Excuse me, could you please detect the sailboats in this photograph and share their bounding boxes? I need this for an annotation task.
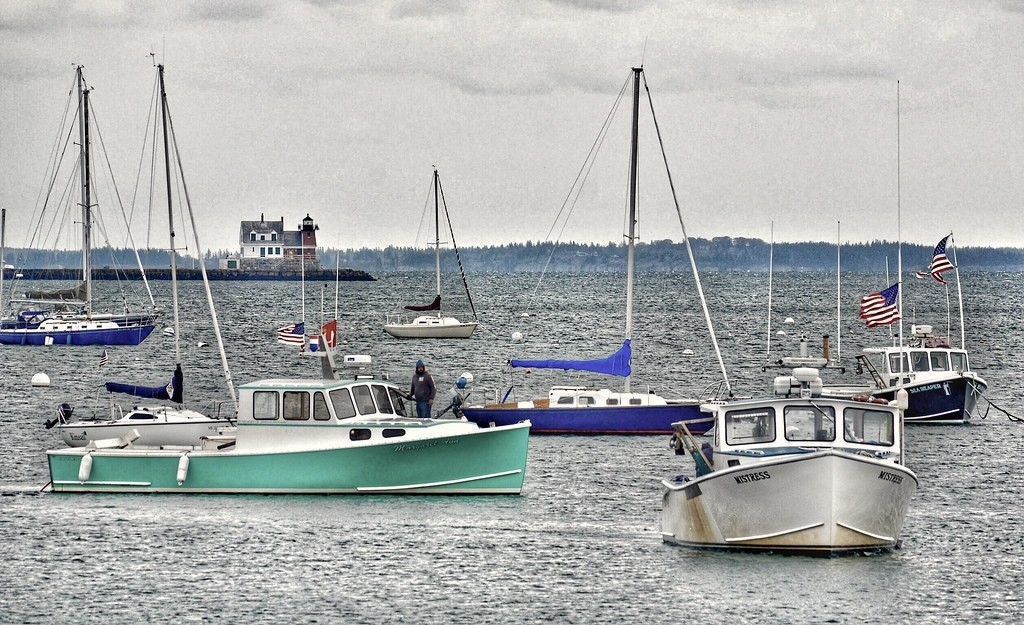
[44,49,535,496]
[383,165,479,339]
[0,62,164,347]
[821,78,988,425]
[298,232,338,358]
[456,34,750,437]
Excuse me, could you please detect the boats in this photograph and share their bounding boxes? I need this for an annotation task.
[661,218,920,562]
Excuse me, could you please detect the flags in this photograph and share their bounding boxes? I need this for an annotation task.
[99,350,108,368]
[322,319,336,348]
[277,322,305,345]
[915,235,956,284]
[860,283,902,328]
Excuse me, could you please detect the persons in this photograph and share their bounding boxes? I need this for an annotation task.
[407,360,436,418]
